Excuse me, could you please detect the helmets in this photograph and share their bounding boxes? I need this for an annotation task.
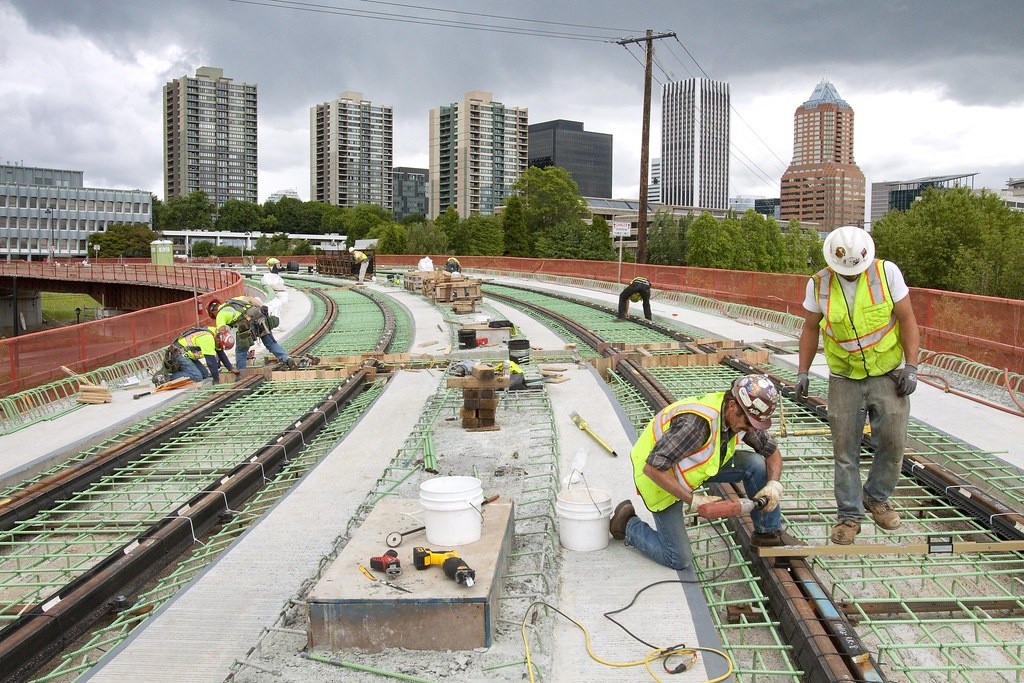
[205,298,221,319]
[733,373,779,430]
[214,330,235,351]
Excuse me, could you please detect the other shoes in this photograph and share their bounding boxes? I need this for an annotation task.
[644,319,653,325]
[612,317,626,322]
[152,368,170,389]
[286,358,300,371]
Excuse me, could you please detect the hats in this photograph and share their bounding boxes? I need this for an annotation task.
[823,226,875,275]
[349,247,355,253]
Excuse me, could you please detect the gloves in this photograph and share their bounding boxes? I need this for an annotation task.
[895,363,919,396]
[795,371,809,404]
[753,480,784,513]
[689,494,723,513]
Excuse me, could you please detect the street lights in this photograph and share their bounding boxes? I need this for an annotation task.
[238,239,243,257]
[74,308,82,324]
[186,242,192,258]
[45,206,55,265]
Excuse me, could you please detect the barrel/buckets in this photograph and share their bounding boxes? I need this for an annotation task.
[555,466,612,552]
[508,340,530,365]
[418,476,485,547]
[458,329,476,350]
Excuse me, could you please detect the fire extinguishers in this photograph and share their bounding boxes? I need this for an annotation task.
[197,296,203,315]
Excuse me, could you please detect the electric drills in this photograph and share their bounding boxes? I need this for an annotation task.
[413,546,477,588]
[698,497,769,521]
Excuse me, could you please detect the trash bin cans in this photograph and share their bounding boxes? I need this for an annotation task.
[150,240,175,266]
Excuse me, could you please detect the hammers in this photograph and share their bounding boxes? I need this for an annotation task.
[133,392,150,400]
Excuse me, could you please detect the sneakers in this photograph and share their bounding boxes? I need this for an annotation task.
[862,498,900,530]
[608,499,636,540]
[830,519,861,545]
[749,525,810,560]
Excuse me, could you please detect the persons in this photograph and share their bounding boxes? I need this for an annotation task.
[610,374,809,571]
[349,247,369,285]
[153,327,241,386]
[266,257,280,274]
[206,296,298,371]
[445,253,462,272]
[611,277,653,322]
[794,226,921,545]
